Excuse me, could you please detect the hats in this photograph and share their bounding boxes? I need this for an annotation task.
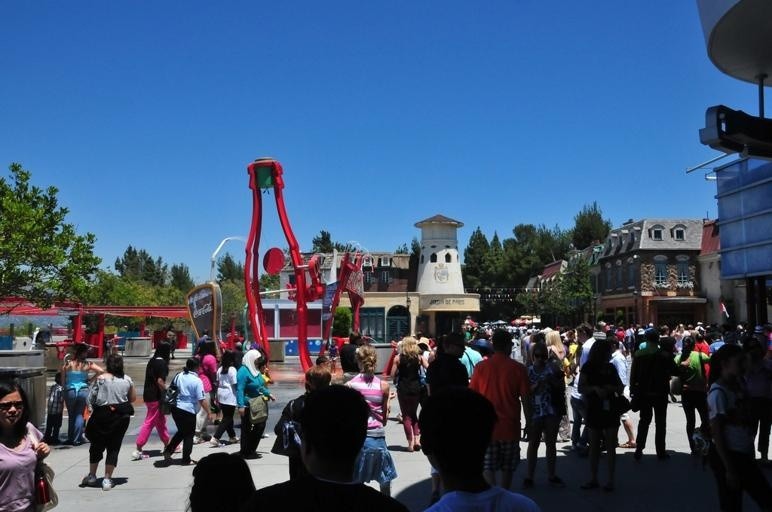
[418,337,432,351]
[697,322,703,324]
[34,439,58,512]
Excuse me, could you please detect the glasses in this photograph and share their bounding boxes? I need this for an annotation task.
[0,402,24,410]
[535,353,548,359]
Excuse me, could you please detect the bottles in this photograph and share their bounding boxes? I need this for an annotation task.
[36,473,49,504]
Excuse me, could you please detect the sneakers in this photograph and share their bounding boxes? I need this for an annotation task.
[521,479,534,489]
[261,433,269,437]
[658,455,671,459]
[228,436,241,444]
[210,437,225,447]
[549,475,562,483]
[131,452,148,461]
[102,479,114,490]
[163,445,181,453]
[82,474,97,485]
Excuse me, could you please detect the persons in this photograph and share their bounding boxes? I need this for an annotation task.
[0,374,50,512]
[183,451,259,511]
[257,313,771,511]
[78,355,137,491]
[30,322,257,464]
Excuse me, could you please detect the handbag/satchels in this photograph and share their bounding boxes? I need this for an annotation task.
[270,399,296,457]
[163,372,181,409]
[670,377,681,395]
[692,389,728,457]
[586,396,630,418]
[248,395,268,423]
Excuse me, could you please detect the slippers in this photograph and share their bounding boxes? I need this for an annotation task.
[620,442,637,448]
